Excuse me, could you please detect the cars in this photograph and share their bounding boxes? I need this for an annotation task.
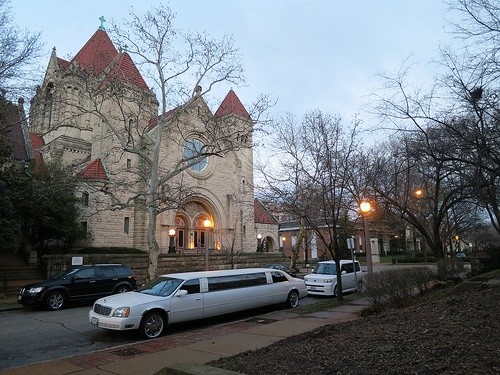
[303,260,363,298]
[265,264,296,282]
[88,268,308,339]
[454,252,467,262]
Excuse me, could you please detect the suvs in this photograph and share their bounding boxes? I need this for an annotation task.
[17,264,138,311]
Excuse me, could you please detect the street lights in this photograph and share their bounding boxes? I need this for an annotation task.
[204,220,211,271]
[282,236,286,254]
[359,201,374,296]
